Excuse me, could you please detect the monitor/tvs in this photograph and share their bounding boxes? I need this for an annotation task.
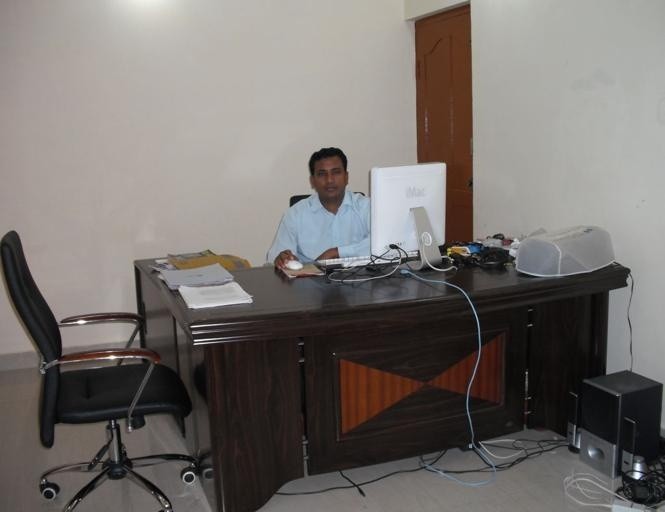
[369,161,448,271]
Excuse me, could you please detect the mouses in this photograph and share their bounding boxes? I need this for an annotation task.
[284,259,304,271]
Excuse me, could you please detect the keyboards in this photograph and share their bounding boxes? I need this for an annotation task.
[317,256,399,267]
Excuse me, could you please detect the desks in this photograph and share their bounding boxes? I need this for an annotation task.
[133,257,629,512]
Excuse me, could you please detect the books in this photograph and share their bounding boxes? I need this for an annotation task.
[150,244,254,309]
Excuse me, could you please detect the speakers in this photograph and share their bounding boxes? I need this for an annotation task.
[566,391,581,453]
[621,416,649,481]
[579,369,664,478]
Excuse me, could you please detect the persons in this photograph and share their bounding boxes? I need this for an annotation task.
[265,147,371,268]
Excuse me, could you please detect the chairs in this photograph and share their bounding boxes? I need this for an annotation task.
[0,230,198,512]
[290,193,365,209]
